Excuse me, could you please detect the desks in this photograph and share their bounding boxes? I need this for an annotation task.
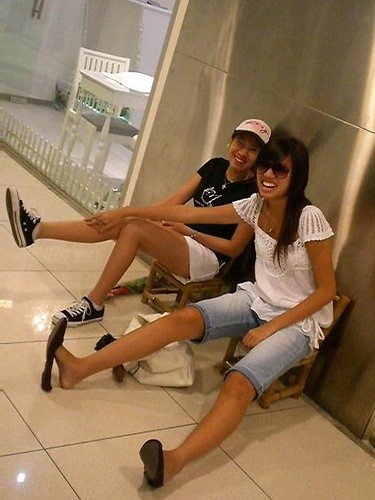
[64,69,150,174]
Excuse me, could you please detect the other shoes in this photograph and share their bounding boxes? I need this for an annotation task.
[40,317,68,392]
[139,439,164,488]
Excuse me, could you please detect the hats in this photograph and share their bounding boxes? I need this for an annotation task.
[231,118,272,145]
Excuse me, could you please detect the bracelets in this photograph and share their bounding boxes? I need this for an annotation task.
[190,231,198,237]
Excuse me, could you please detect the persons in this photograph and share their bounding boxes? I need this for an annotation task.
[41,136,336,488]
[6,118,272,327]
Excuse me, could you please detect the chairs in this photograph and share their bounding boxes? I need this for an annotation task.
[58,46,138,169]
[140,257,237,315]
[221,294,351,409]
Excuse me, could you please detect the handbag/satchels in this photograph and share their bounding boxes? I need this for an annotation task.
[120,311,196,388]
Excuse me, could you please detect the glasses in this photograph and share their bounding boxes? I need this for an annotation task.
[253,161,289,179]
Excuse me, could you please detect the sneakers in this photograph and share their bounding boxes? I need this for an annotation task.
[6,187,42,249]
[52,296,105,328]
